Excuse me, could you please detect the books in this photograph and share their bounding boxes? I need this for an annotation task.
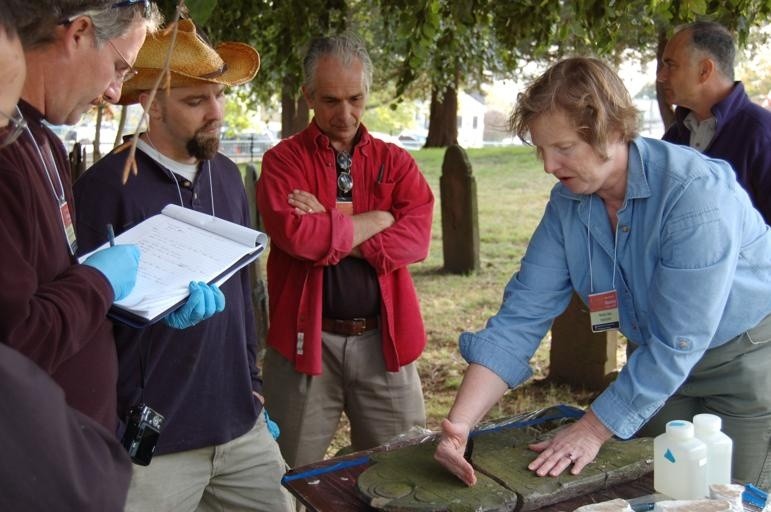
[78,201,271,330]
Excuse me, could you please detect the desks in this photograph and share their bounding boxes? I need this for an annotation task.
[280,406,654,512]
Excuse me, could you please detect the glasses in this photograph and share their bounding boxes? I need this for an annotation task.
[55,12,143,87]
[335,149,355,194]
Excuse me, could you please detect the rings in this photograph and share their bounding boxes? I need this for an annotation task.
[564,452,576,463]
[308,208,314,213]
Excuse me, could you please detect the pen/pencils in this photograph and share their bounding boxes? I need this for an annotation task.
[107,223,115,247]
[377,164,384,184]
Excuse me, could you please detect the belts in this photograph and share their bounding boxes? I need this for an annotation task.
[323,316,378,336]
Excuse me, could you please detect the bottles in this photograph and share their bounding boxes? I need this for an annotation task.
[653,419,709,501]
[692,413,733,485]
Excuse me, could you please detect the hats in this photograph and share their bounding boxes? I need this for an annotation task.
[102,15,264,108]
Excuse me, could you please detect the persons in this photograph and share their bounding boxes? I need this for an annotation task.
[0,0,227,447]
[73,18,299,511]
[659,21,771,228]
[254,32,434,467]
[0,11,29,130]
[435,58,770,495]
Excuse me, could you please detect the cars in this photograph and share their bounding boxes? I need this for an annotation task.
[397,129,428,146]
[220,126,274,158]
[64,128,125,163]
